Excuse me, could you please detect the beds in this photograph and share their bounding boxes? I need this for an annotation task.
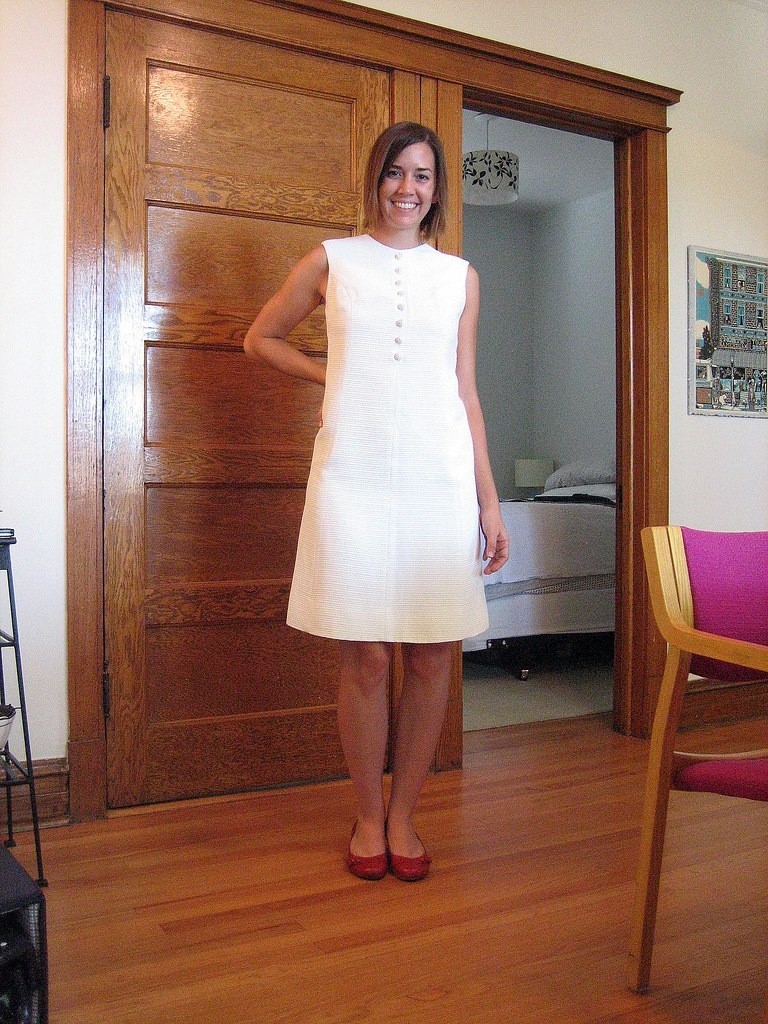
[462,457,616,682]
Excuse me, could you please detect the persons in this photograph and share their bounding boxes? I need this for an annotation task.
[243,121,511,883]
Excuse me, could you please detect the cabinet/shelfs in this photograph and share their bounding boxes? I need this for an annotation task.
[0,528,49,888]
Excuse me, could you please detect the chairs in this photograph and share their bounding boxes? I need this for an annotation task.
[623,524,768,993]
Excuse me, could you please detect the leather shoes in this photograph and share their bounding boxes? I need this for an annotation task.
[383,815,440,882]
[347,822,389,879]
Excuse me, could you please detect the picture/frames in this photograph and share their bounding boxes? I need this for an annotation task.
[686,244,768,419]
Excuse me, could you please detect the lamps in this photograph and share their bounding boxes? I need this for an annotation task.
[462,113,519,206]
[515,459,553,497]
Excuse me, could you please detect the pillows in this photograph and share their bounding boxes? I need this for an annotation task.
[544,458,616,492]
